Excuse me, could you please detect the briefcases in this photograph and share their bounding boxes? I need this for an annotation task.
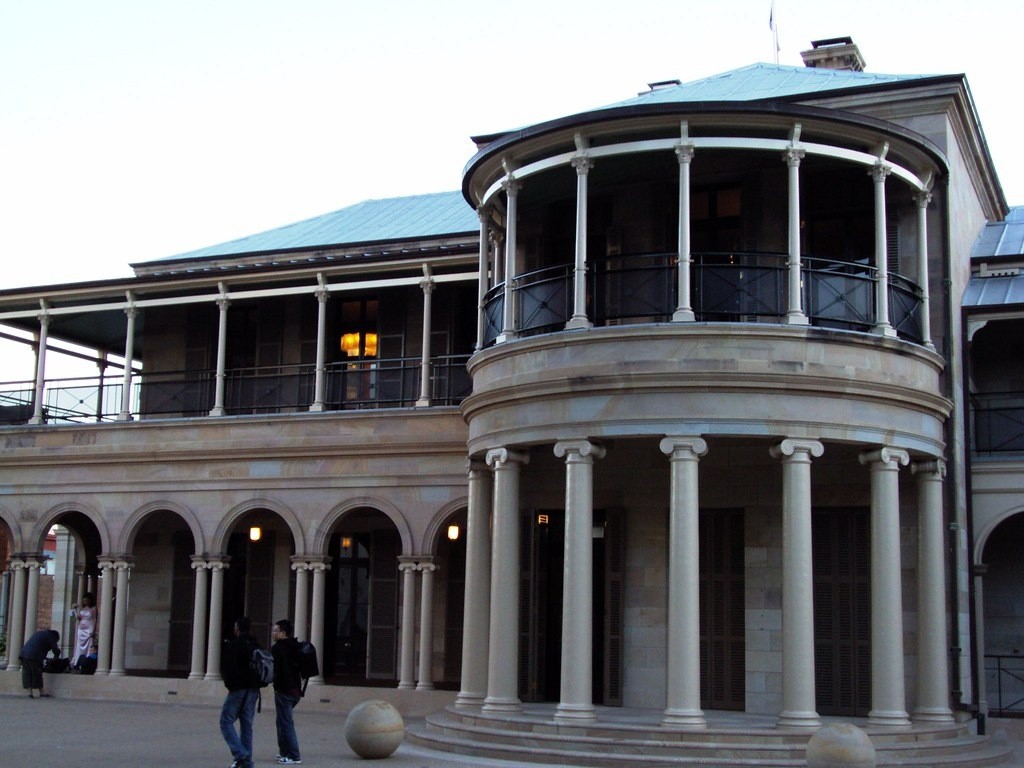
[45,658,71,673]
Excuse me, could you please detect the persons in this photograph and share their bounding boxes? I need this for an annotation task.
[269,618,320,765]
[18,629,61,699]
[219,617,274,768]
[71,585,117,675]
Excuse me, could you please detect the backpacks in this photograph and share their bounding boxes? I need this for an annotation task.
[295,639,319,697]
[240,638,275,713]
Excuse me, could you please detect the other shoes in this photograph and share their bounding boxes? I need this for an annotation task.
[28,695,33,698]
[274,755,282,760]
[279,757,301,764]
[230,753,251,768]
[40,693,53,697]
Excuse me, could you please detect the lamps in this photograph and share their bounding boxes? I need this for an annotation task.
[341,332,379,365]
